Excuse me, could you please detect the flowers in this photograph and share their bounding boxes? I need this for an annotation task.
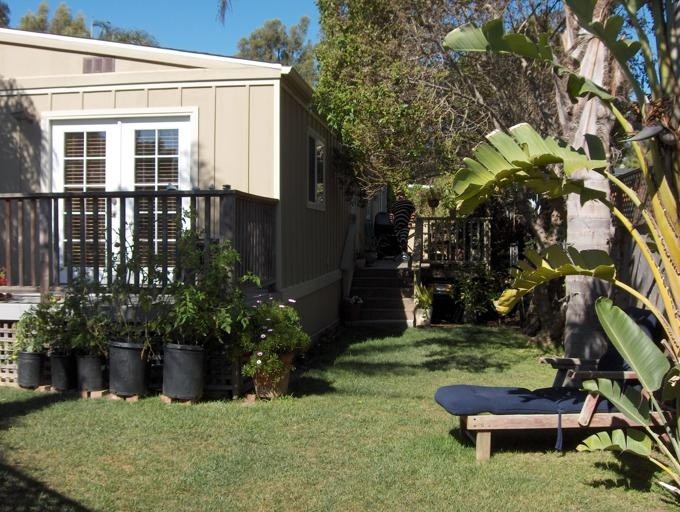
[239,292,311,383]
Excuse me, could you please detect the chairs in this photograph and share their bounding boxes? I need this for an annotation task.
[434,303,673,460]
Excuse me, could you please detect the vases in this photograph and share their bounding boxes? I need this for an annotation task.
[250,349,294,399]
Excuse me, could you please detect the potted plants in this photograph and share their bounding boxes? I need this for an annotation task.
[2,306,50,389]
[426,187,443,207]
[62,245,120,391]
[414,282,432,327]
[102,218,173,396]
[153,206,262,401]
[39,284,84,391]
